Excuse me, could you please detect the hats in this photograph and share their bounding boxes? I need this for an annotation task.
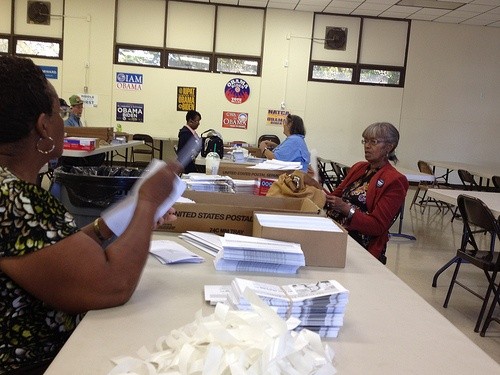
[69,95,83,105]
[59,98,70,107]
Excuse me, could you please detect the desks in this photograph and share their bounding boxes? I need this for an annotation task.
[58,139,148,165]
[426,189,500,223]
[316,152,437,240]
[36,219,499,375]
[194,147,255,165]
[427,158,500,193]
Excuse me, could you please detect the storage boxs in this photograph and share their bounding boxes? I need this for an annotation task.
[158,140,352,268]
[63,126,129,152]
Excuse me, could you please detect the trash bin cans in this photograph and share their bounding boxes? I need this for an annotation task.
[54,165,144,247]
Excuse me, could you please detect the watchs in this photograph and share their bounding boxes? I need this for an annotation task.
[348,205,356,216]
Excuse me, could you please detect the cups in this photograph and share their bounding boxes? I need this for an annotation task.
[205,152,220,175]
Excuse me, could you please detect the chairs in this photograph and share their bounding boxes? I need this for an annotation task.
[130,133,153,164]
[408,160,500,341]
[257,135,282,148]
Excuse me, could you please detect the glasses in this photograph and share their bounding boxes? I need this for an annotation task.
[362,139,389,145]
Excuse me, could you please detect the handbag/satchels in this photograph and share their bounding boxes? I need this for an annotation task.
[266,172,327,209]
[200,136,223,159]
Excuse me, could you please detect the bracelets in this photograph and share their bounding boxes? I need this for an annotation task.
[94,218,111,243]
[262,147,268,155]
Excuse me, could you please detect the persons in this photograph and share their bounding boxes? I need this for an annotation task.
[322,122,409,260]
[176,110,206,174]
[0,54,184,375]
[259,114,310,174]
[47,95,106,172]
